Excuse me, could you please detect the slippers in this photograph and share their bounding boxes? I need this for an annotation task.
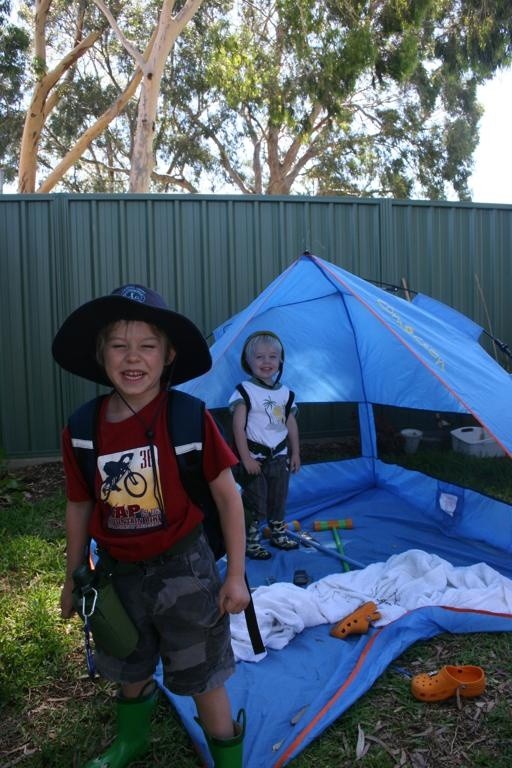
[329,601,383,640]
[410,662,487,703]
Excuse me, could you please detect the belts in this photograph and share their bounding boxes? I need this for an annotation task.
[156,518,205,557]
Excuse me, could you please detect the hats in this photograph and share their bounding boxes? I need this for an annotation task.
[47,279,216,394]
[239,330,286,378]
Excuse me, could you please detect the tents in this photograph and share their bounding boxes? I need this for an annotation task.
[81,247,510,768]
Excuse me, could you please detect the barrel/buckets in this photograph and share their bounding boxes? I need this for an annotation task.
[402,428,424,455]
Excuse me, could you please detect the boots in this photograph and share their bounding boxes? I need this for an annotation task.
[80,689,161,768]
[243,517,272,561]
[267,518,301,551]
[194,716,245,768]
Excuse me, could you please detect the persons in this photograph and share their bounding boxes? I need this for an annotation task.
[226,329,301,561]
[49,280,254,768]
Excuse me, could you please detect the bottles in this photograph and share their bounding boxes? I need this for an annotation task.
[70,565,140,661]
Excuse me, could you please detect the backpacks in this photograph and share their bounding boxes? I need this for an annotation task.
[65,388,252,565]
